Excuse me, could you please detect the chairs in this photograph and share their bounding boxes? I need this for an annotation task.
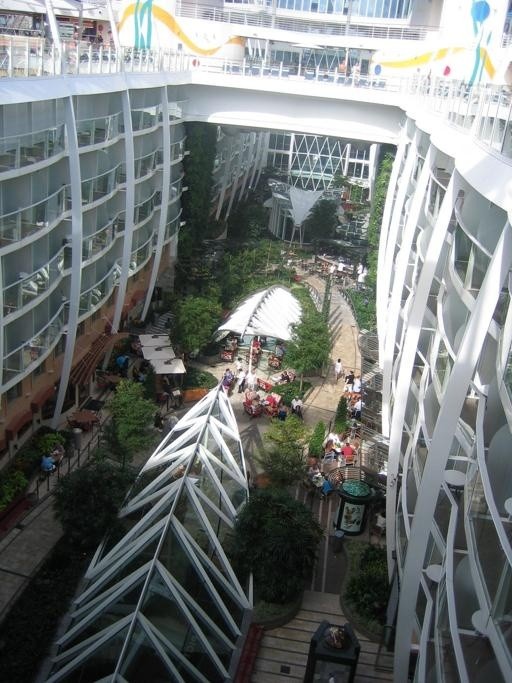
[305,370,360,485]
[221,335,303,421]
[36,353,134,481]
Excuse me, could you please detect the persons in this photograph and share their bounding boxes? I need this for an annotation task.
[339,61,361,84]
[154,411,178,432]
[356,262,364,275]
[220,335,304,424]
[40,441,65,472]
[166,318,172,329]
[321,261,346,274]
[349,423,360,439]
[309,464,332,497]
[117,354,147,382]
[335,359,365,419]
[325,439,355,459]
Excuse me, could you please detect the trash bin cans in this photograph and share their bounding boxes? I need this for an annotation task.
[333,531,344,553]
[73,428,82,450]
